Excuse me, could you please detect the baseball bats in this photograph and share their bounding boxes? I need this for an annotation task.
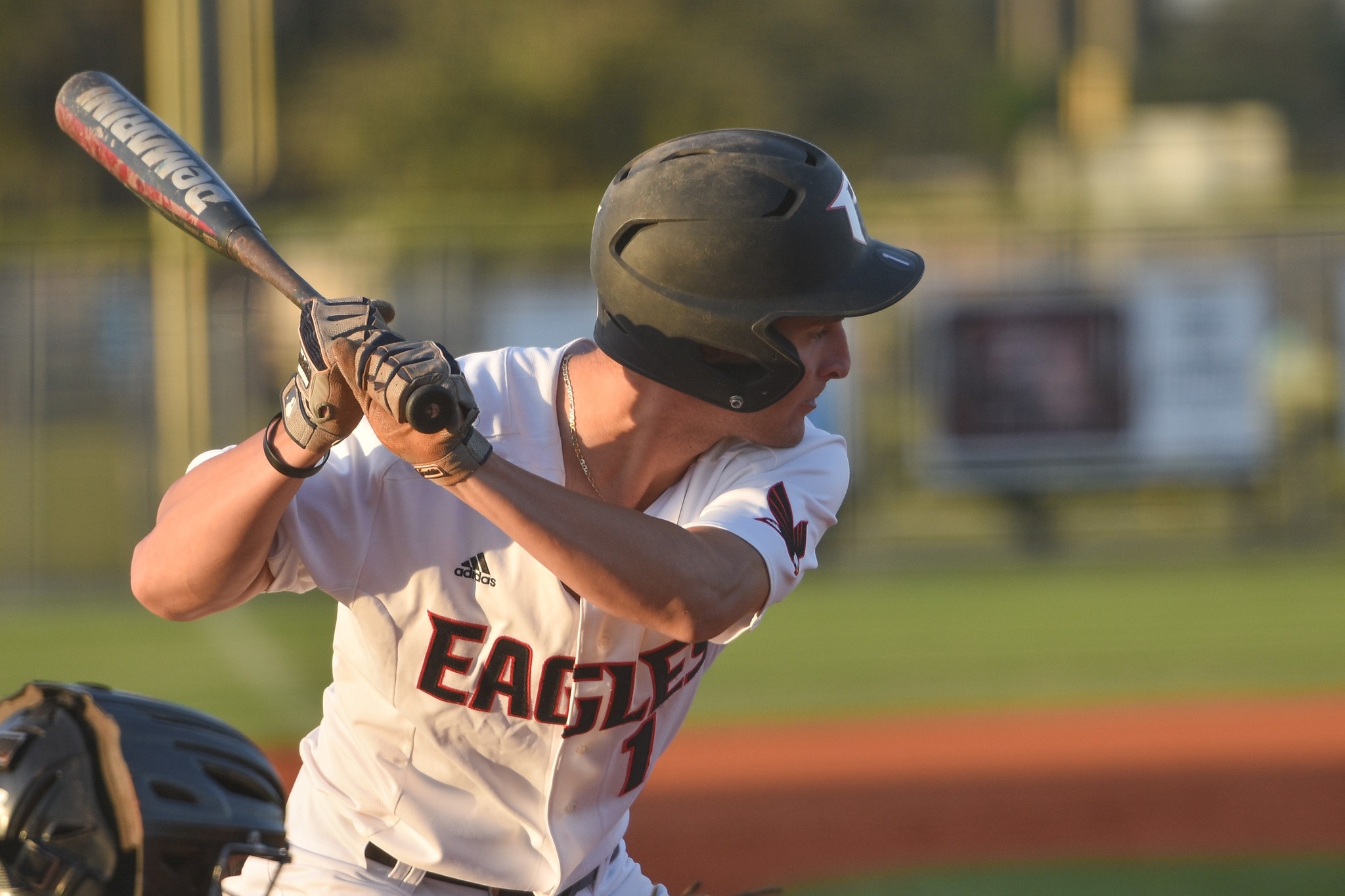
[55,70,456,434]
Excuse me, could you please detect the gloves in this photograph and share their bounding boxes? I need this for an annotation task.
[282,296,405,455]
[331,327,502,491]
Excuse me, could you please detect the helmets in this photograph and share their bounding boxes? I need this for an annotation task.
[0,672,289,896]
[587,130,924,414]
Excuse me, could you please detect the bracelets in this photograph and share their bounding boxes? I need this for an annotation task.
[261,410,331,478]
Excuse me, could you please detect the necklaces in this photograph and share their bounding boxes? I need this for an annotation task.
[562,352,610,501]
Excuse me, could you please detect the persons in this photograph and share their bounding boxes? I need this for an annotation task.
[130,129,927,896]
